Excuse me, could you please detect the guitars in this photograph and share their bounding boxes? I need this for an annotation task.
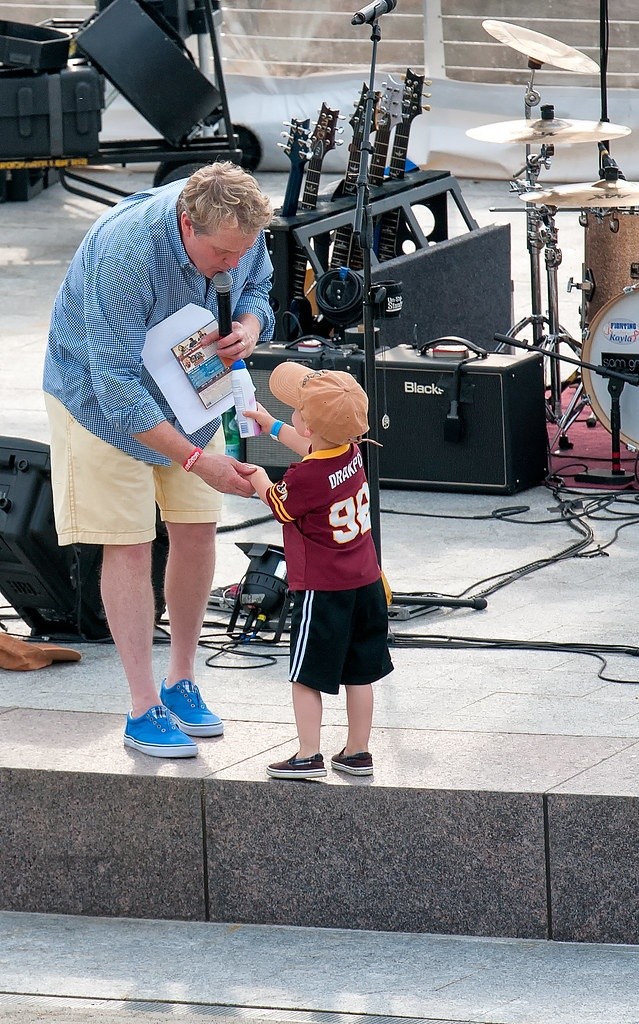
[275,67,433,320]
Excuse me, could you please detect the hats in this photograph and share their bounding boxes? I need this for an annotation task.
[269,362,370,445]
[0,632,81,671]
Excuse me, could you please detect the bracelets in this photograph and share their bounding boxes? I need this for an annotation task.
[270,420,284,443]
[183,447,204,473]
[178,330,209,369]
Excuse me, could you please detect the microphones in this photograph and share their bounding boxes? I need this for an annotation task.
[213,272,233,339]
[351,0,397,25]
[444,369,462,442]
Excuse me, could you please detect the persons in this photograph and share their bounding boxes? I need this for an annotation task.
[237,361,395,779]
[43,161,278,758]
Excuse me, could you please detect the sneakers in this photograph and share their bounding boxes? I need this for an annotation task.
[159,677,224,737]
[331,747,373,776]
[124,706,198,757]
[266,751,327,779]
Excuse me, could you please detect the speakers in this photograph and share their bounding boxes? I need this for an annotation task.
[0,434,169,641]
[240,340,549,494]
[74,0,223,148]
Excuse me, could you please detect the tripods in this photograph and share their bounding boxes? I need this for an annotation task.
[494,53,586,423]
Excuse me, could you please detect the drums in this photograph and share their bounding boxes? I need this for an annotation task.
[581,205,639,331]
[580,282,639,453]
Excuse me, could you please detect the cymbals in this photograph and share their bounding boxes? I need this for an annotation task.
[481,18,604,75]
[517,181,639,208]
[462,117,634,146]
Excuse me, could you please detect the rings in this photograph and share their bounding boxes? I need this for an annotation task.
[239,341,246,348]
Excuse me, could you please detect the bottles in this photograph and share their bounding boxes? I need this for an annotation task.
[231,359,260,438]
